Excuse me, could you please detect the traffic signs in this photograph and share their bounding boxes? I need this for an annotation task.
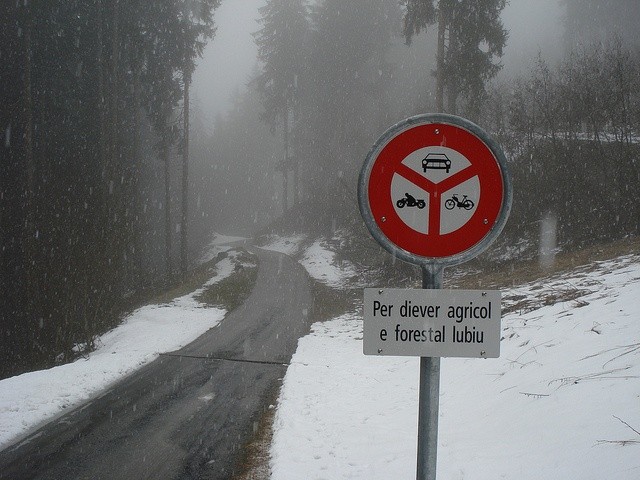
[363,288,501,358]
